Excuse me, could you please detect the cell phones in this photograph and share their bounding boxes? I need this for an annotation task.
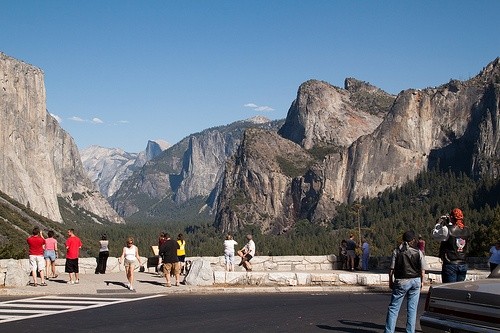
[440,215,447,220]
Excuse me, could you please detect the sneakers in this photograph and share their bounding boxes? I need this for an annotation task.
[41,282,47,286]
[67,280,74,285]
[74,279,80,284]
[34,283,37,287]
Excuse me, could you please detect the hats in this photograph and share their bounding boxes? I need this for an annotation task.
[418,234,422,238]
[494,239,500,244]
[402,231,415,242]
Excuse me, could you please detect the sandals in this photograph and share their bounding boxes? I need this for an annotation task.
[52,274,58,278]
[46,277,49,280]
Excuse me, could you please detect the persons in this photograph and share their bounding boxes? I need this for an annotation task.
[223,235,238,272]
[346,234,357,271]
[44,231,58,280]
[159,232,166,250]
[339,240,348,270]
[432,208,470,283]
[384,231,425,333]
[95,234,110,274]
[362,239,370,271]
[237,234,255,271]
[119,238,143,291]
[26,228,48,287]
[417,234,425,255]
[488,240,500,274]
[177,233,187,275]
[65,229,83,284]
[159,233,180,287]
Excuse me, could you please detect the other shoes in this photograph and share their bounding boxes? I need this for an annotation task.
[239,258,248,266]
[341,265,370,275]
[175,282,179,286]
[166,283,171,287]
[128,283,133,290]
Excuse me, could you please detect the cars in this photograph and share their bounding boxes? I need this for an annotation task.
[418,264,500,333]
